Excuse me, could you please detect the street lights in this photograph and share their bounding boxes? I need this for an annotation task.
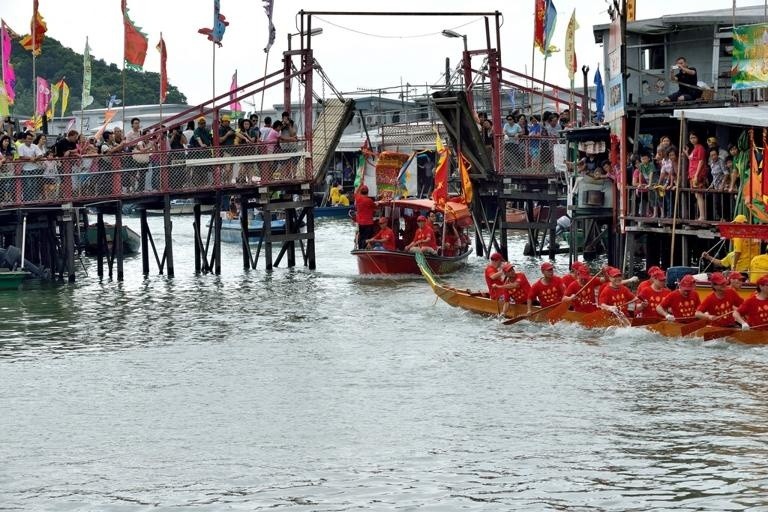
[283,26,326,141]
[438,28,472,107]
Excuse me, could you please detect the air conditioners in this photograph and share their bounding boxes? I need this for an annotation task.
[365,115,377,126]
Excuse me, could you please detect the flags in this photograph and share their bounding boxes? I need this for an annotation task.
[156,41,168,104]
[564,9,576,77]
[198,1,230,46]
[262,0,277,52]
[20,7,49,57]
[229,74,241,112]
[120,1,150,73]
[430,132,472,204]
[83,45,94,107]
[535,1,559,53]
[34,76,69,113]
[2,23,17,105]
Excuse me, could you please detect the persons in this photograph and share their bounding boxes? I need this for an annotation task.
[1,111,302,195]
[330,180,470,255]
[478,56,768,333]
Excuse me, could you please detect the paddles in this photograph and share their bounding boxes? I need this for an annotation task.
[631,317,697,327]
[583,296,638,330]
[503,302,563,327]
[681,311,733,337]
[704,323,768,342]
[548,271,602,326]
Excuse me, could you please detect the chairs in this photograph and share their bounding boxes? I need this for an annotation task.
[659,89,712,106]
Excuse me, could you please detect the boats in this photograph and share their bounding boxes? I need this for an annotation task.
[0,216,38,292]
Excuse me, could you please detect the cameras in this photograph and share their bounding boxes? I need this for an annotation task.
[175,127,182,134]
[288,117,294,125]
[672,66,679,69]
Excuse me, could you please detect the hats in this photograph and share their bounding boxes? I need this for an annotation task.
[490,253,768,290]
[706,136,717,145]
[196,113,234,124]
[360,185,426,225]
